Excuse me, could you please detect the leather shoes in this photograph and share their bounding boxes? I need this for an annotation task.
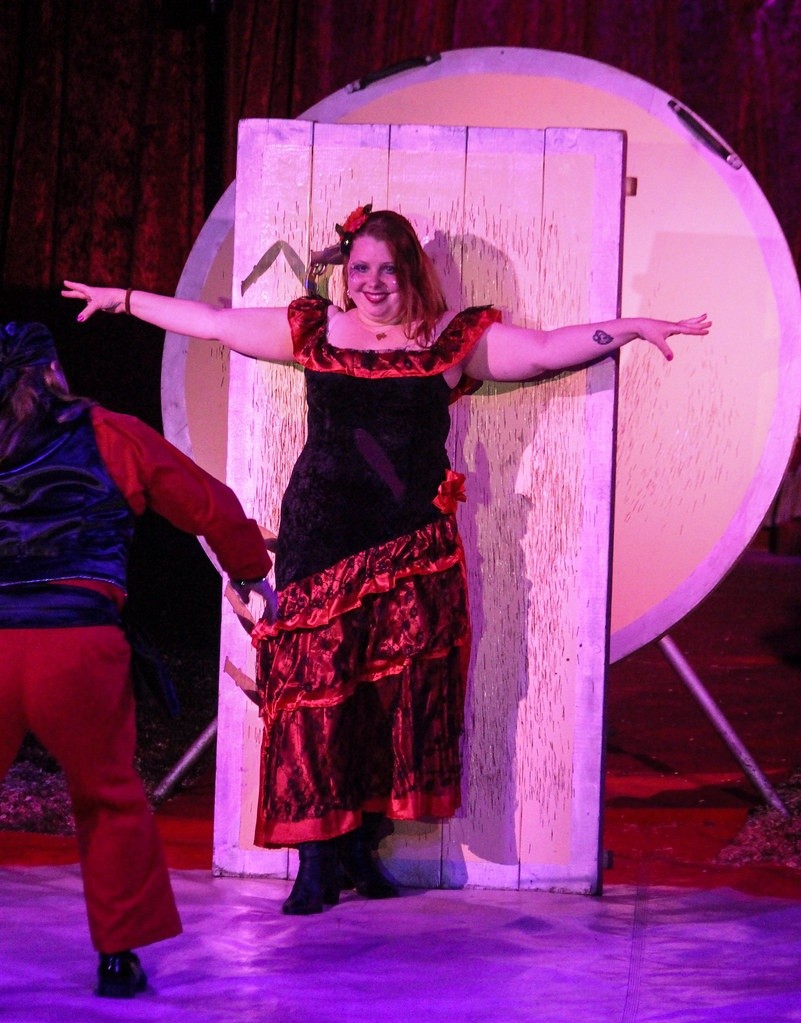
[96,952,147,998]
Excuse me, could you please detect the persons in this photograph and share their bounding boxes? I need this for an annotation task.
[60,203,713,916]
[0,323,272,995]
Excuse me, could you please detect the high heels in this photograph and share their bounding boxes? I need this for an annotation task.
[344,827,397,899]
[282,838,343,914]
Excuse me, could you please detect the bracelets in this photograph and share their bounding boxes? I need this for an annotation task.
[239,577,266,586]
[125,288,132,315]
